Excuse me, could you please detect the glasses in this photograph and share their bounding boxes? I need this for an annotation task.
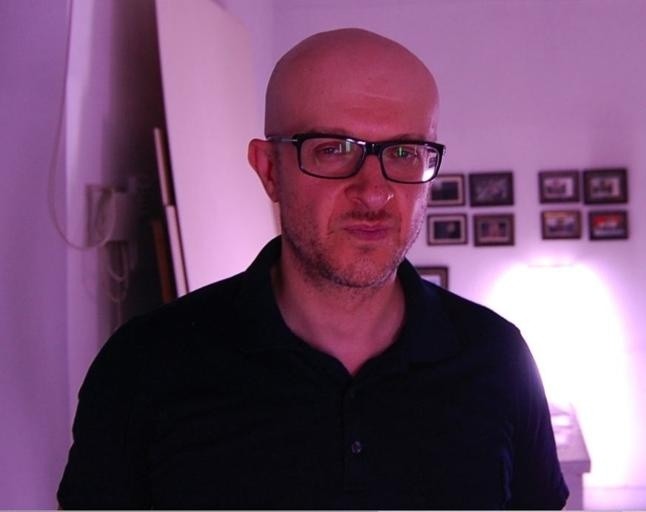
[267,133,447,184]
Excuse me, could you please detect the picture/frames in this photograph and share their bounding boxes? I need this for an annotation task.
[414,266,448,292]
[427,166,630,246]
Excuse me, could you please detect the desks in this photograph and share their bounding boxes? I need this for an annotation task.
[546,401,590,511]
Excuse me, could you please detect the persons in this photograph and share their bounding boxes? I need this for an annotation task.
[57,29,572,508]
